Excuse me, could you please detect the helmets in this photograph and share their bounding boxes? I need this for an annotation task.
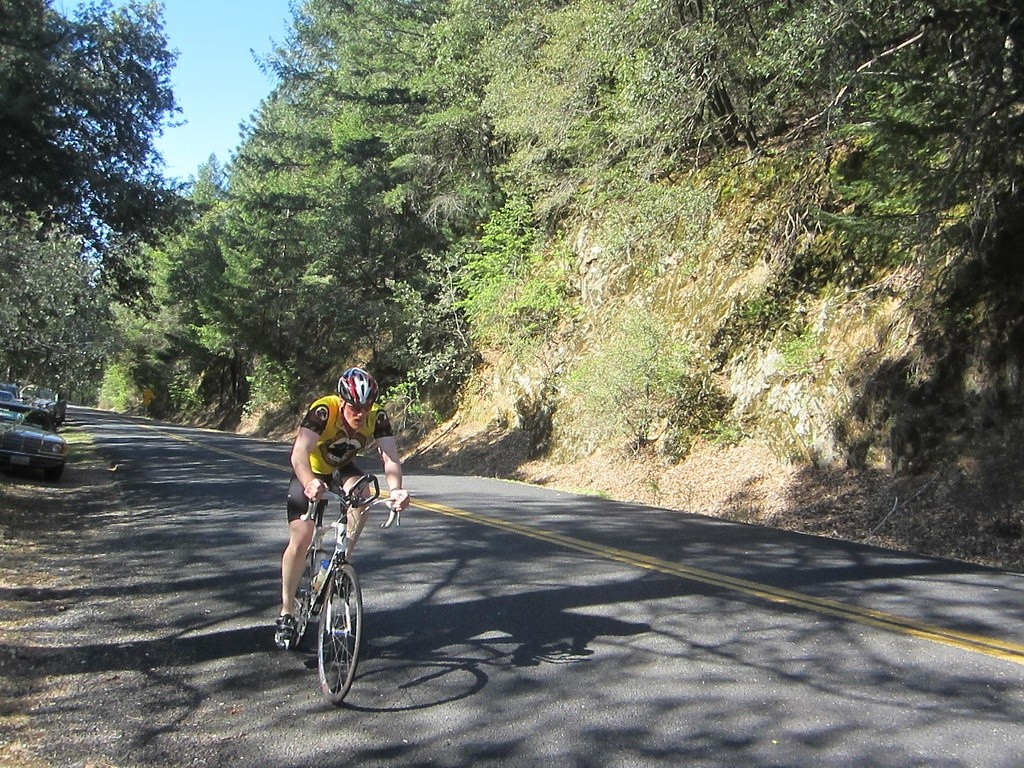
[338,367,379,412]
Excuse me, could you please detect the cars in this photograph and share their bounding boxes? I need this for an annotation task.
[0,378,67,428]
[0,401,71,483]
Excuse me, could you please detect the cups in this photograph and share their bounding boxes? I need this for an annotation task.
[313,559,331,596]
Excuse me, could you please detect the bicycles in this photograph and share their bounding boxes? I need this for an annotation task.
[278,472,406,705]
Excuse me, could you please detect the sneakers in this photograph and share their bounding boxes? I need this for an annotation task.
[274,615,297,649]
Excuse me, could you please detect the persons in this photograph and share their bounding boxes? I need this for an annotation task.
[272,368,410,649]
